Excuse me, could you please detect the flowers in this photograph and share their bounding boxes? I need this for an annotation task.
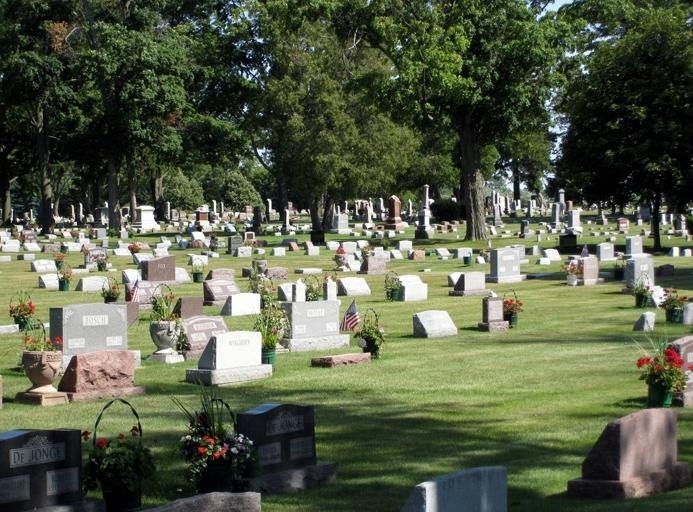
[659,289,688,313]
[631,328,693,392]
[166,375,261,484]
[79,426,156,492]
[252,310,284,352]
[58,268,72,285]
[94,254,109,269]
[102,278,121,300]
[18,318,62,352]
[151,284,175,322]
[323,272,339,282]
[54,253,65,266]
[352,310,385,352]
[632,283,654,299]
[11,290,35,325]
[503,298,524,315]
[191,258,206,273]
[250,272,273,309]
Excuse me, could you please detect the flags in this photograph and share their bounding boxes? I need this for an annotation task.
[339,299,360,333]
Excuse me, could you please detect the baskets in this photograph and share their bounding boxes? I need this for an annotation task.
[102,277,119,302]
[197,398,237,493]
[646,384,672,408]
[262,349,274,372]
[634,274,649,308]
[57,262,71,291]
[93,399,141,512]
[9,291,32,331]
[362,308,377,358]
[191,258,203,282]
[666,286,681,323]
[503,289,517,329]
